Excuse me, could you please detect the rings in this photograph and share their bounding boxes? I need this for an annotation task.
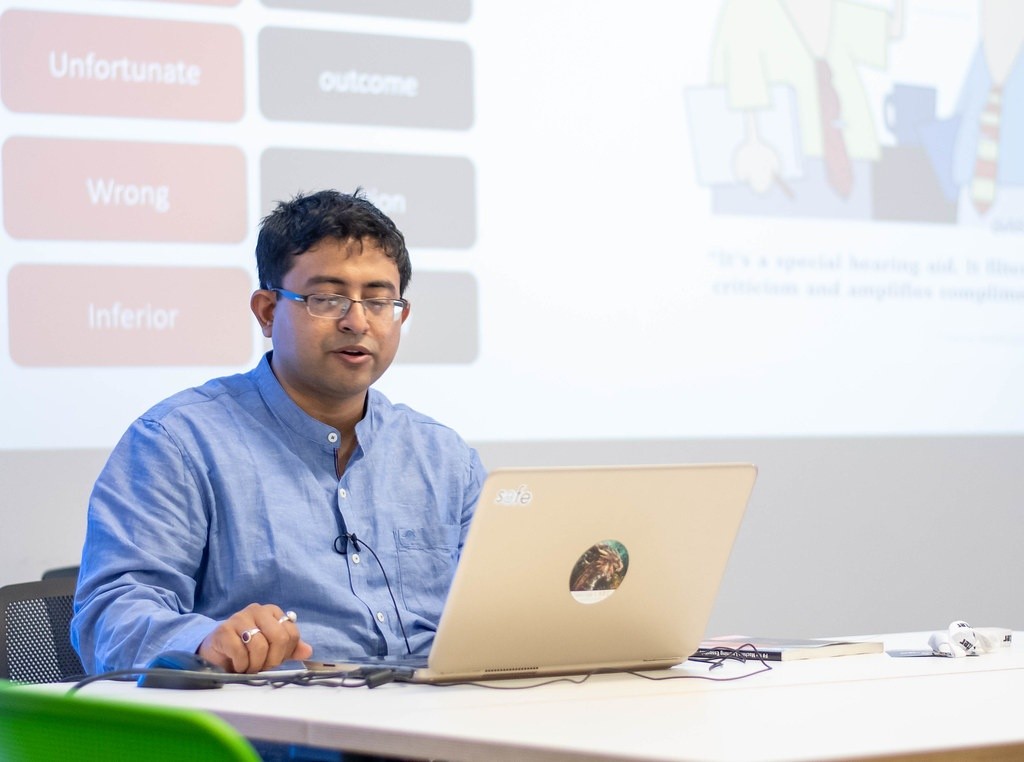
[278,610,297,623]
[241,628,260,643]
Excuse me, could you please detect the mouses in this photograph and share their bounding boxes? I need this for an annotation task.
[135,650,227,690]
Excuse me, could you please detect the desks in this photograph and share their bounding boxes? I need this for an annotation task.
[20,624,1024,762]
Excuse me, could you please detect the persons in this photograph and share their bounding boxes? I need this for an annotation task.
[67,190,486,682]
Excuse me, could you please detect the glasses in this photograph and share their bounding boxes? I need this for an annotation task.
[268,287,407,326]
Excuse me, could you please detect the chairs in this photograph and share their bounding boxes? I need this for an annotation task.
[0,677,262,762]
[0,577,86,685]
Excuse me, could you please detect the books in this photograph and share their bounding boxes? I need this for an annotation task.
[690,634,884,662]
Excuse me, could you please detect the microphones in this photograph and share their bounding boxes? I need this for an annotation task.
[350,533,361,552]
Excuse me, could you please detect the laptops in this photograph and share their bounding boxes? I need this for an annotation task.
[297,463,758,684]
